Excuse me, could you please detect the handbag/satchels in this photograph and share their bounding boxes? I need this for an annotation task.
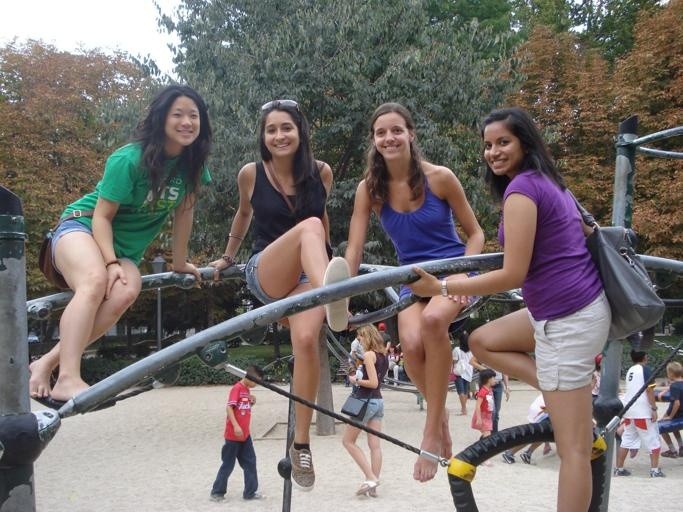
[38,237,70,289]
[448,374,457,380]
[587,226,665,340]
[341,396,367,421]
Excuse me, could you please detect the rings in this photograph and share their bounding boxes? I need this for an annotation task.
[214,269,220,273]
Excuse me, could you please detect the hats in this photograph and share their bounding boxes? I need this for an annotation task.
[379,323,386,331]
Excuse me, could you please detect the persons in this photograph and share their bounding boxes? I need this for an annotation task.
[405,106,613,512]
[208,363,263,503]
[207,98,352,493]
[592,350,683,478]
[452,330,511,439]
[503,395,555,464]
[28,85,212,401]
[345,102,486,482]
[341,322,390,498]
[352,331,403,386]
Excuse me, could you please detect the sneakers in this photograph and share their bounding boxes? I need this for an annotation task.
[210,495,228,502]
[323,257,351,332]
[502,447,682,477]
[289,440,315,492]
[250,493,267,499]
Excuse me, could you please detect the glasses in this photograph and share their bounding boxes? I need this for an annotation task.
[261,99,300,112]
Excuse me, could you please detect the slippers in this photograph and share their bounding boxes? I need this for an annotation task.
[357,480,380,499]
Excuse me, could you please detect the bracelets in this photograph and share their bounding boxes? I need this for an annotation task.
[104,260,121,270]
[221,255,235,265]
[227,233,245,241]
[440,280,448,297]
